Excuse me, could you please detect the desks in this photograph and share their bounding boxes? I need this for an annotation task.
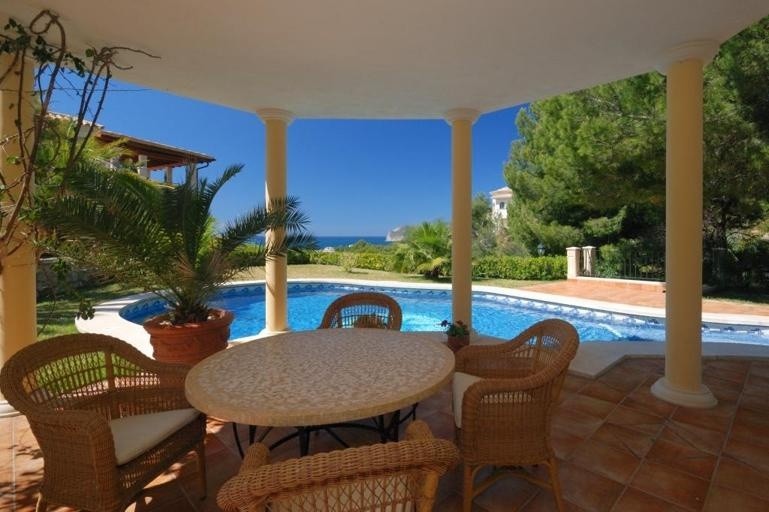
[180,324,458,457]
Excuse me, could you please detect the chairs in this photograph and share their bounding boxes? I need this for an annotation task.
[0,289,578,511]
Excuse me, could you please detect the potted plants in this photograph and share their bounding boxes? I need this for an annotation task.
[15,143,324,370]
